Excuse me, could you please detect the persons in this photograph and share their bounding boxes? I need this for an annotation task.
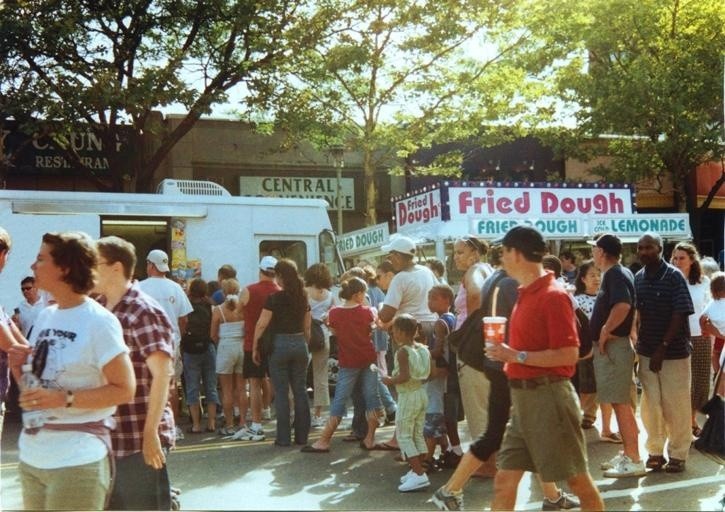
[378,237,441,342]
[430,267,521,511]
[207,281,219,297]
[543,256,596,426]
[452,236,497,478]
[302,263,334,424]
[380,314,431,491]
[348,267,366,282]
[252,259,312,448]
[132,247,194,393]
[428,286,464,464]
[182,279,221,436]
[377,260,395,291]
[92,236,176,510]
[559,252,579,281]
[484,226,605,511]
[209,279,248,436]
[573,259,622,444]
[700,277,725,396]
[298,278,378,453]
[592,235,647,478]
[1,227,33,448]
[206,265,237,305]
[8,231,137,512]
[364,266,396,422]
[13,278,49,337]
[633,235,694,475]
[425,258,450,289]
[669,242,713,437]
[702,258,720,275]
[238,255,283,442]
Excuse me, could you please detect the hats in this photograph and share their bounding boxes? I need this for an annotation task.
[585,233,622,255]
[487,225,546,256]
[258,255,278,273]
[380,236,416,256]
[146,248,171,273]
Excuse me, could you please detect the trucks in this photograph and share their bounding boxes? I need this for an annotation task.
[0,180,348,397]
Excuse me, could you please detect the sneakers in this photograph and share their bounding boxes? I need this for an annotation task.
[600,450,624,470]
[541,488,581,511]
[665,457,686,472]
[310,415,323,427]
[261,409,272,420]
[646,456,667,470]
[232,424,266,441]
[445,449,464,468]
[432,484,465,512]
[603,456,647,478]
[400,468,413,484]
[398,471,431,492]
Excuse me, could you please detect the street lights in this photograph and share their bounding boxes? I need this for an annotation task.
[323,142,354,237]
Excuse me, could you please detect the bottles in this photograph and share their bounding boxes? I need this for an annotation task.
[19,364,44,429]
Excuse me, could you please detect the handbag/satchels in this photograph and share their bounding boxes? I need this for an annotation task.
[257,292,277,360]
[448,272,509,372]
[693,355,725,465]
[308,316,326,353]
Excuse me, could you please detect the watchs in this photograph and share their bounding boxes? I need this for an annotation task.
[517,350,529,364]
[64,389,74,407]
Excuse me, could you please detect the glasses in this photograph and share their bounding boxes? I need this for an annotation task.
[21,286,33,291]
[375,274,381,280]
[456,234,479,249]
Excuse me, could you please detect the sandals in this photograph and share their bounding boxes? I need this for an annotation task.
[218,425,235,435]
[692,424,702,438]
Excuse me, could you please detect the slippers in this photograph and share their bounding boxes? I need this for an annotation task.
[187,426,202,434]
[300,445,330,453]
[342,434,364,441]
[376,442,400,450]
[599,433,623,443]
[359,439,376,449]
